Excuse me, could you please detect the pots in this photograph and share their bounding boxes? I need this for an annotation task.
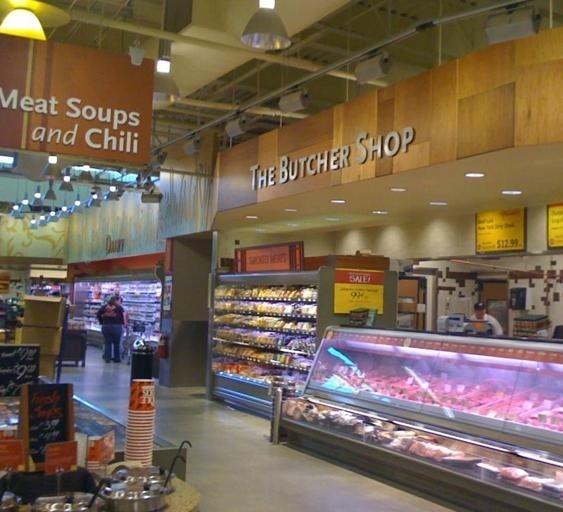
[0,464,178,512]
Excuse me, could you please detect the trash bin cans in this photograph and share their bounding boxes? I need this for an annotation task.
[130,349,153,381]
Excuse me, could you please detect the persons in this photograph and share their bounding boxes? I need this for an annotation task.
[101,297,127,360]
[462,301,504,336]
[96,296,127,364]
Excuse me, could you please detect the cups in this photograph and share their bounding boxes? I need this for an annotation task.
[123,379,158,469]
[85,436,107,485]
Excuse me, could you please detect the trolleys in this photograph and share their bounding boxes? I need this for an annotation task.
[122,325,148,365]
[59,330,88,368]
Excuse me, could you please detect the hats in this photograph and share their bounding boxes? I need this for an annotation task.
[474,303,484,309]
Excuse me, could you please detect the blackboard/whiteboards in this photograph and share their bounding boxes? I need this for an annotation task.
[23,383,75,472]
[0,343,41,397]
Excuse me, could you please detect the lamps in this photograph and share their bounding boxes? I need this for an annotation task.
[147,2,544,167]
[9,167,163,230]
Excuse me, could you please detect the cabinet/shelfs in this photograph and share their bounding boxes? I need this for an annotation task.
[81,273,172,359]
[278,324,563,511]
[210,270,398,421]
